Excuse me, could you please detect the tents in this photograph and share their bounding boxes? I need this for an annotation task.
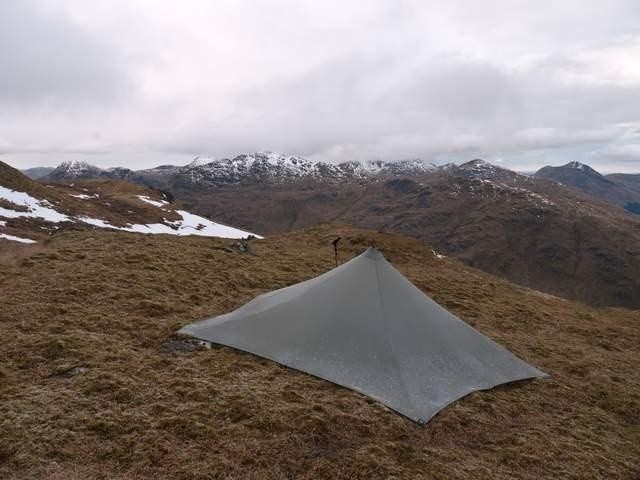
[176,245,552,428]
